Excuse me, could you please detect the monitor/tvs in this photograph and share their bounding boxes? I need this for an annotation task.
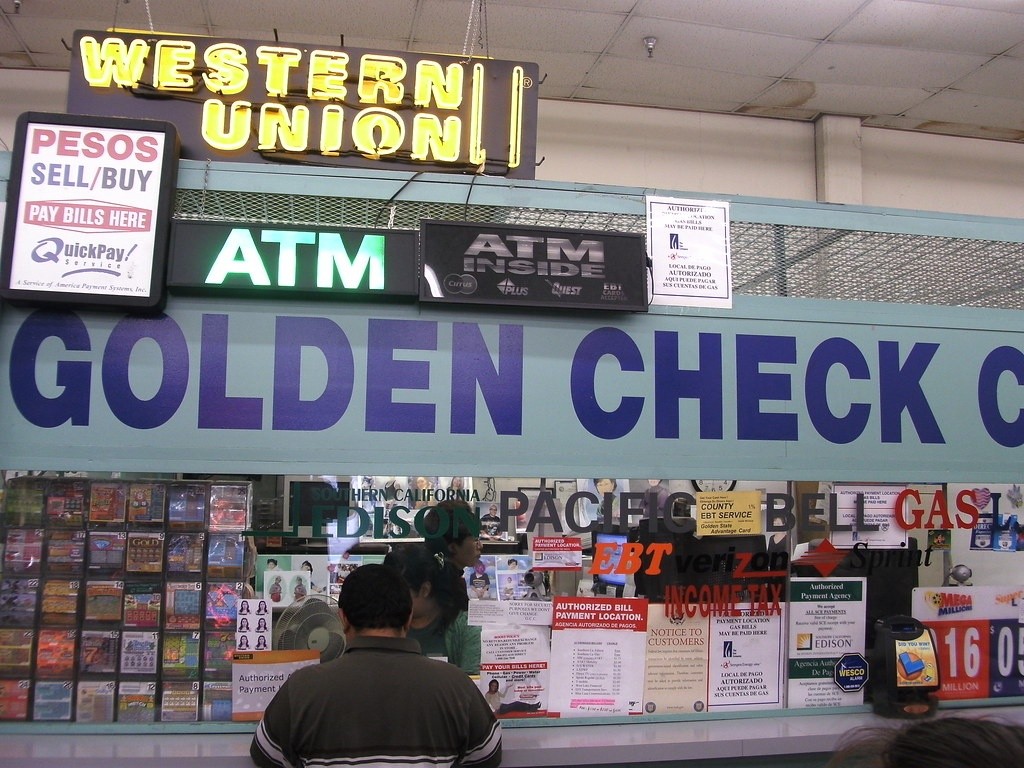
[592,531,629,586]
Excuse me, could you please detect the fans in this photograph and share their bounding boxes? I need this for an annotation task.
[269,593,348,665]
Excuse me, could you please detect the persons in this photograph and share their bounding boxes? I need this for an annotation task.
[235,479,671,652]
[483,680,542,715]
[249,565,503,768]
[830,715,1023,768]
[384,544,481,673]
[425,500,485,611]
